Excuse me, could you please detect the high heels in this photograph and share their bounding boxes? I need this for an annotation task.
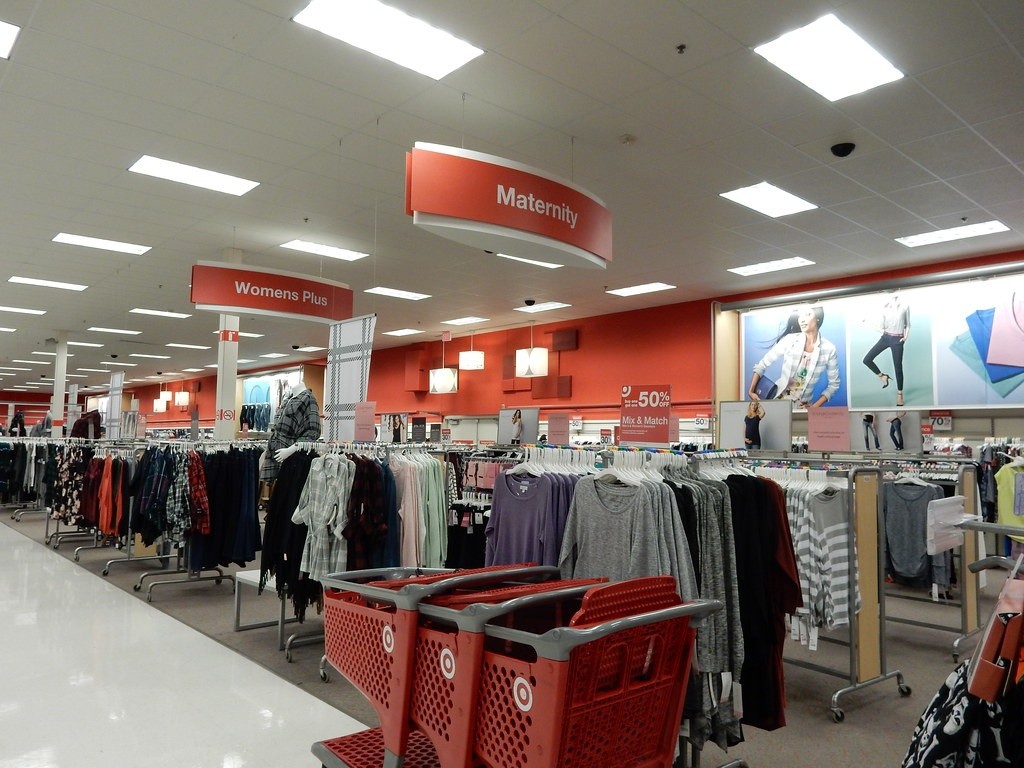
[896,394,903,406]
[879,373,893,389]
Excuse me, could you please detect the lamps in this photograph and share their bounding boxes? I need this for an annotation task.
[459,331,485,370]
[174,378,190,406]
[515,320,549,378]
[153,382,166,412]
[429,335,458,394]
[160,381,172,402]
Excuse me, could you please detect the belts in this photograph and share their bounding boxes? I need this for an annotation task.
[884,332,903,337]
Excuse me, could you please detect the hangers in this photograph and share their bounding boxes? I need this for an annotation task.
[452,489,495,511]
[933,437,968,453]
[692,450,757,482]
[751,466,843,497]
[593,451,688,489]
[295,441,436,464]
[0,436,89,448]
[145,439,269,456]
[976,435,1024,468]
[91,447,137,461]
[880,461,961,489]
[504,447,600,478]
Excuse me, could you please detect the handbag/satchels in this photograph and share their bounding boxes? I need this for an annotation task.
[754,375,777,400]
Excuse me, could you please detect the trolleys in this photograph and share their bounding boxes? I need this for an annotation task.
[310,564,725,768]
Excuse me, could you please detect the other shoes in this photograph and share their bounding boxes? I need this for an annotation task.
[877,448,881,450]
[867,449,870,451]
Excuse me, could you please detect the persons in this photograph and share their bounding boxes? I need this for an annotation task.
[29,419,41,437]
[240,402,271,432]
[861,413,881,450]
[42,410,51,438]
[742,402,765,450]
[259,382,320,482]
[863,293,911,407]
[393,415,402,442]
[8,410,24,437]
[511,410,522,444]
[886,412,906,450]
[749,307,840,407]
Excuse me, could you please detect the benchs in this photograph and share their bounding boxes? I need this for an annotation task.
[235,569,326,651]
